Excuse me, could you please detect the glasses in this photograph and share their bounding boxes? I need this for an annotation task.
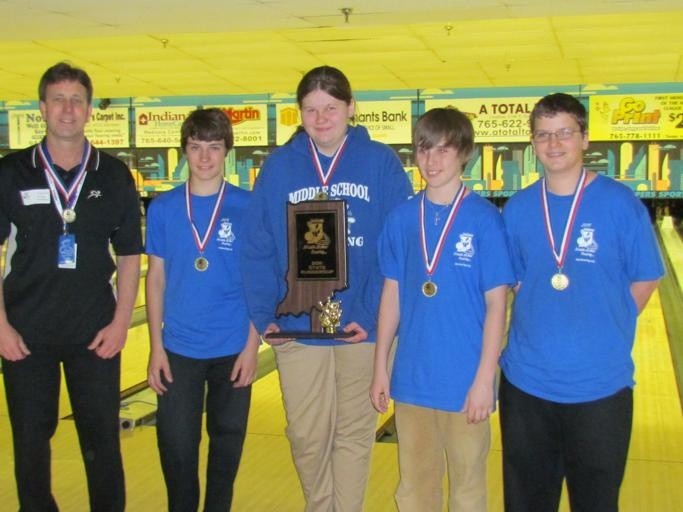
[534,127,582,143]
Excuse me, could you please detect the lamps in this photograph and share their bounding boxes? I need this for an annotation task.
[161,39,168,47]
[339,6,351,23]
[444,23,454,35]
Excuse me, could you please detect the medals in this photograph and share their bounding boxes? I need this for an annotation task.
[310,189,328,200]
[61,207,77,225]
[193,255,209,273]
[420,279,437,299]
[549,272,569,291]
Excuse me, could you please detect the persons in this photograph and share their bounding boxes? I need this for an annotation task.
[240,64,414,511]
[367,107,517,511]
[2,61,143,512]
[141,107,262,512]
[498,91,667,511]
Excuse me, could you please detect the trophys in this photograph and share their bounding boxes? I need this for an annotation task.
[263,198,358,341]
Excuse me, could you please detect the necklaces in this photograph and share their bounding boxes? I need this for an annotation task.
[432,201,450,226]
